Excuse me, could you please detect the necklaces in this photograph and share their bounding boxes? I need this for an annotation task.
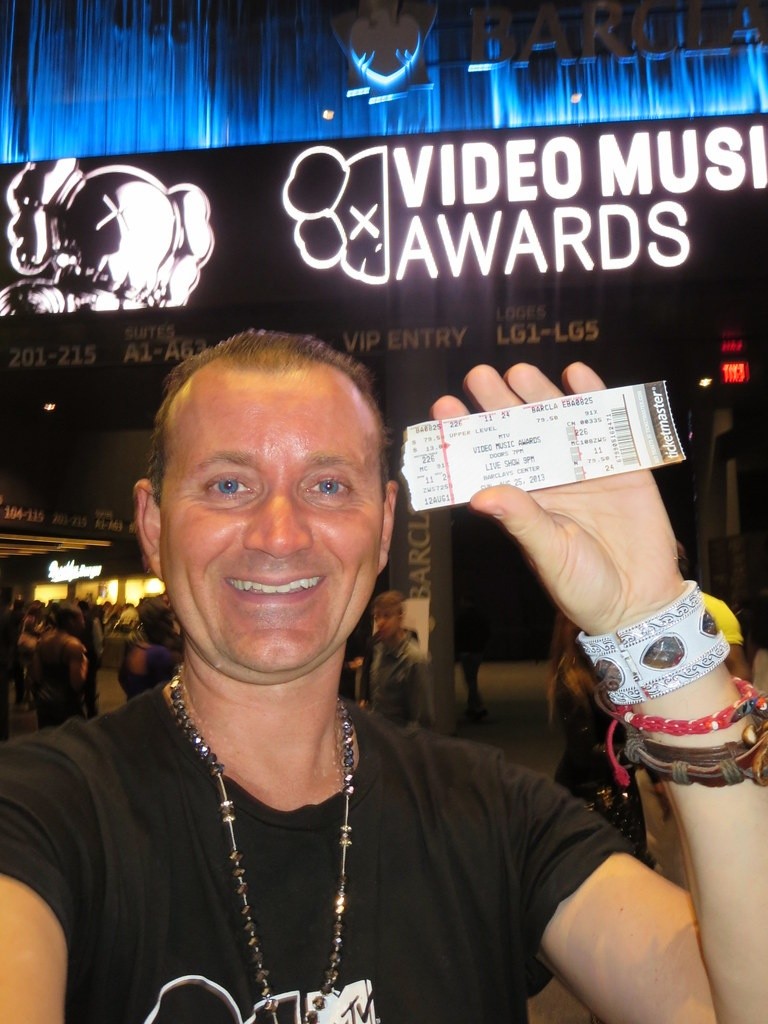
[167,672,356,1024]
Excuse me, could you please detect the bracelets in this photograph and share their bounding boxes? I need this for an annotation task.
[575,582,729,704]
[618,713,767,788]
[606,676,757,787]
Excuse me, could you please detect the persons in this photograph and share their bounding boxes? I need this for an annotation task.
[348,591,430,724]
[1,586,185,730]
[454,590,493,716]
[1,331,768,1024]
[544,533,768,870]
